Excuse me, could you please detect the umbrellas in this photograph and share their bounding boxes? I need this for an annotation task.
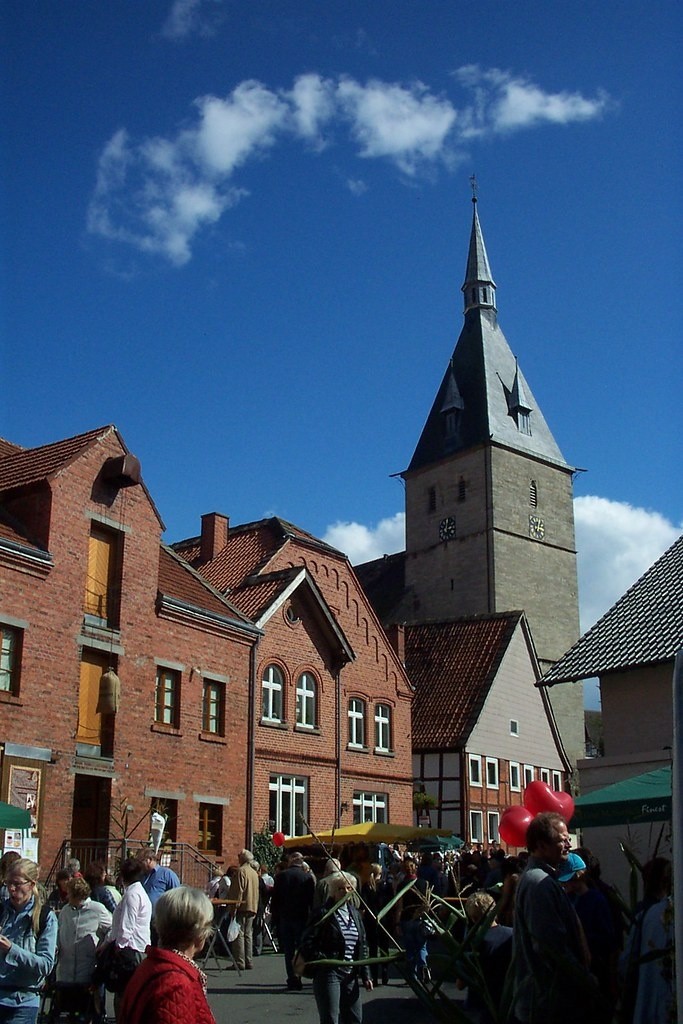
[408,834,465,852]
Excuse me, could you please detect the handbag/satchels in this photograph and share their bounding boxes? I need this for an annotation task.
[292,949,316,979]
[89,941,136,993]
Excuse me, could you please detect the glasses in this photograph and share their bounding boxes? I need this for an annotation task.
[3,880,29,886]
[336,886,353,892]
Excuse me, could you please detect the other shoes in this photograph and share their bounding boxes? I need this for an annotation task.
[253,949,261,956]
[289,976,304,990]
[226,965,254,971]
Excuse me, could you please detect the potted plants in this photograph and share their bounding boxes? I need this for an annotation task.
[413,792,437,812]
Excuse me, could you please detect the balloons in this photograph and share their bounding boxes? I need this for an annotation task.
[524,781,565,816]
[504,805,529,813]
[273,832,284,847]
[552,791,575,825]
[498,813,533,847]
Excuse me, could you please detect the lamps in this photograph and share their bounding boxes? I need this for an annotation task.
[341,800,349,814]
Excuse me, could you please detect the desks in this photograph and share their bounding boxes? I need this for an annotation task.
[199,898,248,978]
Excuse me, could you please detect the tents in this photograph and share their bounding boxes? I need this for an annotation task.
[284,822,452,844]
[567,765,672,828]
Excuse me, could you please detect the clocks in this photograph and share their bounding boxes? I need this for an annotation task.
[437,514,456,543]
[528,514,546,542]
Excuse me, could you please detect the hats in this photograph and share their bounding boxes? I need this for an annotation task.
[288,852,303,866]
[558,853,587,882]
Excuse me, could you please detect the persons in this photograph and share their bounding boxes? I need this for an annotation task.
[0,813,677,1024]
